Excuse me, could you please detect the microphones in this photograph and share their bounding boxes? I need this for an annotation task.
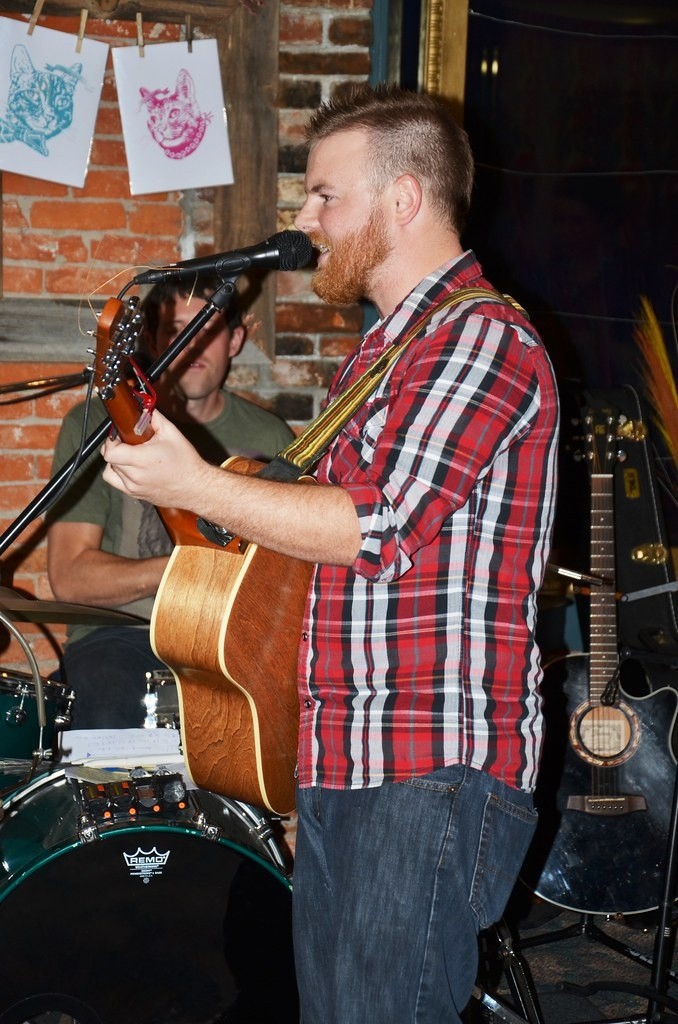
[82,351,155,381]
[132,230,313,285]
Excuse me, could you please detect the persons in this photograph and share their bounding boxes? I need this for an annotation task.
[47,273,297,732]
[97,81,565,1024]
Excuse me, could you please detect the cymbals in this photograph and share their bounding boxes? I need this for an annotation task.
[0,584,152,626]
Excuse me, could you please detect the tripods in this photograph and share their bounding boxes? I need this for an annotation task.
[511,914,678,986]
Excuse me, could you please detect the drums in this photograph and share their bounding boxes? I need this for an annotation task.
[1,768,303,1024]
[0,664,78,796]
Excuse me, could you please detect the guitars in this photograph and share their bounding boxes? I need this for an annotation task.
[82,298,321,815]
[513,397,676,918]
